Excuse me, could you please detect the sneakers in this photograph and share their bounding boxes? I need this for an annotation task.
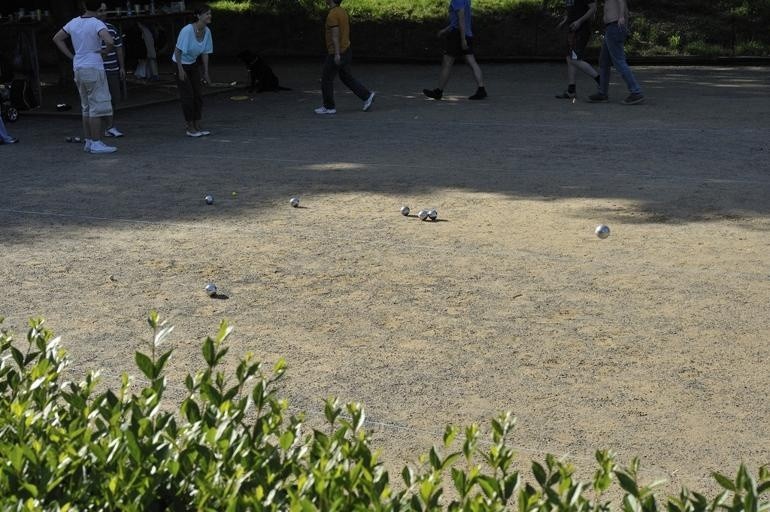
[423,88,444,100]
[468,87,488,100]
[555,91,647,105]
[83,127,126,154]
[186,130,211,137]
[362,91,376,112]
[314,106,336,114]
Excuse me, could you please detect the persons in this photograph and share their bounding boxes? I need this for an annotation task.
[171,4,213,136]
[135,17,160,82]
[53,1,118,155]
[0,113,21,145]
[98,2,127,138]
[554,2,600,99]
[135,38,146,79]
[423,0,487,101]
[587,0,646,105]
[314,0,376,114]
